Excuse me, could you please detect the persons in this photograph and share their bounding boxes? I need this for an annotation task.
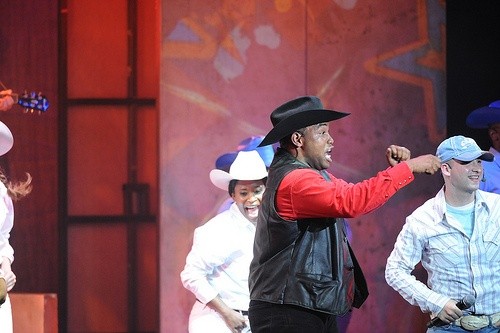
[0,89,33,333]
[385,100,500,333]
[179,135,280,333]
[248,96,442,333]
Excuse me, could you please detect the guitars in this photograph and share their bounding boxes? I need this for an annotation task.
[0,86,51,116]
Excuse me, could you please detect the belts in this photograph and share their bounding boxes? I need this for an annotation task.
[235,310,249,315]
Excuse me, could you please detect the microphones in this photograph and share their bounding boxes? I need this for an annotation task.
[427,294,476,328]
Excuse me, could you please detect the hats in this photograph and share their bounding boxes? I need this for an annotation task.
[210,150,268,191]
[216,136,276,173]
[256,96,351,147]
[466,101,500,129]
[436,135,495,162]
